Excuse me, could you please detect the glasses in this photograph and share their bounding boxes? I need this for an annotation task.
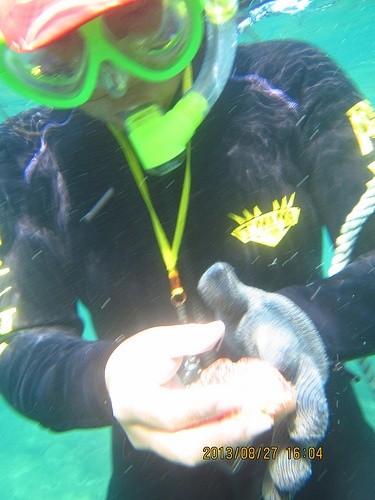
[1,0,206,110]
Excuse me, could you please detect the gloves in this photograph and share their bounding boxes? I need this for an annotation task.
[196,260,332,500]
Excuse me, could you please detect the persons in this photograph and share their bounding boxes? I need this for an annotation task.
[0,1,375,499]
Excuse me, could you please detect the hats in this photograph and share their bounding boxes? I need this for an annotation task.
[1,1,145,53]
